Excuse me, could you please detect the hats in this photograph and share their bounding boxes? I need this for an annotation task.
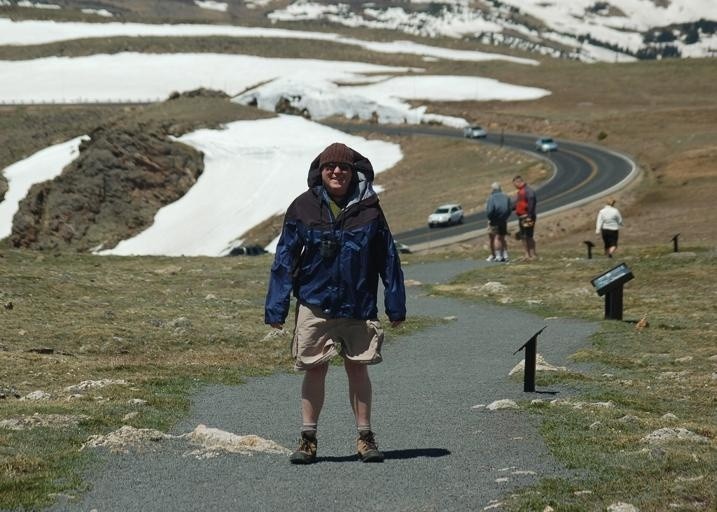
[319,143,354,168]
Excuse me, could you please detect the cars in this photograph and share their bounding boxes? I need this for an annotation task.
[392,238,411,253]
[463,126,487,139]
[535,137,557,153]
[226,245,269,258]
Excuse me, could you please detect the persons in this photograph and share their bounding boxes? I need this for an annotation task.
[485,183,512,262]
[595,199,623,257]
[510,176,538,262]
[265,142,406,463]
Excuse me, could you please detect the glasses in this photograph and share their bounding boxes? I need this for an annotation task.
[324,162,348,170]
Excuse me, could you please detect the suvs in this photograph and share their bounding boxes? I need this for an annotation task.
[427,204,464,228]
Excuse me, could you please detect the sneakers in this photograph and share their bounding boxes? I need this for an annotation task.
[486,254,510,262]
[290,438,317,464]
[357,436,385,462]
